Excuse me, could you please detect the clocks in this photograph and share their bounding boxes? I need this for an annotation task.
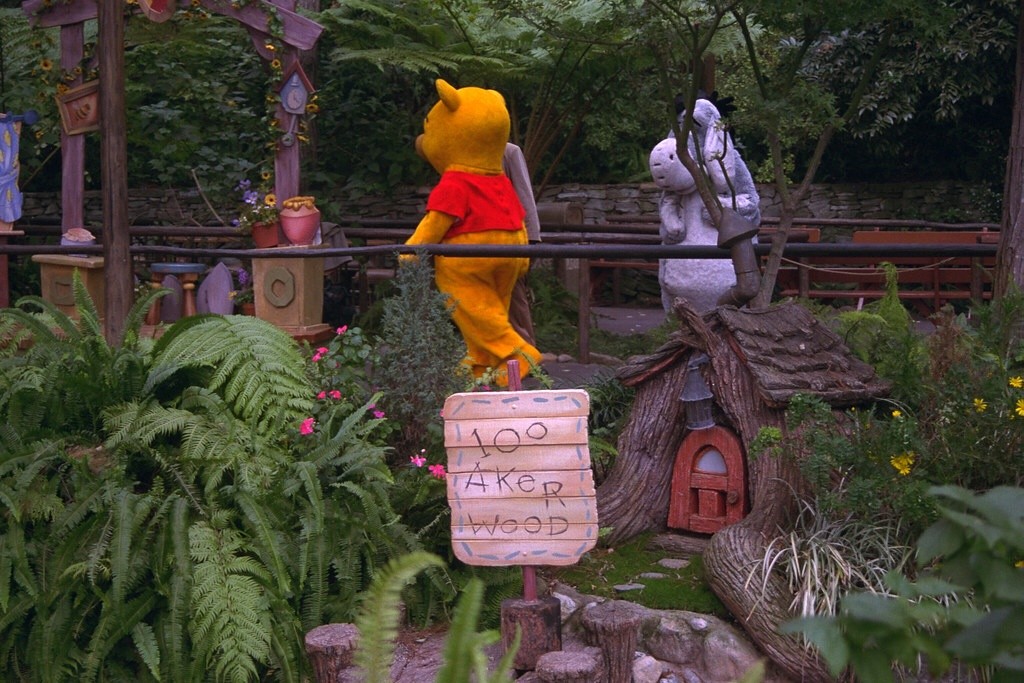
[277,59,315,147]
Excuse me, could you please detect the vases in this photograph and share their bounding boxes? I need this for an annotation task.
[251,221,279,248]
[280,196,321,246]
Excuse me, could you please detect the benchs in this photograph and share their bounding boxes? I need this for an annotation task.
[587,226,1003,328]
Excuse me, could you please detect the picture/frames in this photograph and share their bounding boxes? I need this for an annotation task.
[54,79,101,135]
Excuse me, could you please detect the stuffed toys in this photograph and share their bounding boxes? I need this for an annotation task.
[398,79,542,387]
[649,99,760,311]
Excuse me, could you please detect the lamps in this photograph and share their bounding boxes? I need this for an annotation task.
[680,354,714,430]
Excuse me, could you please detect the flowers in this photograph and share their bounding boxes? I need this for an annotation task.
[32,0,319,151]
[232,168,281,234]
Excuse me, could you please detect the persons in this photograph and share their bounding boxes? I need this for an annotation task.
[503,144,540,343]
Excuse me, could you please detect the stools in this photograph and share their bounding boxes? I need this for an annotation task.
[146,263,206,325]
